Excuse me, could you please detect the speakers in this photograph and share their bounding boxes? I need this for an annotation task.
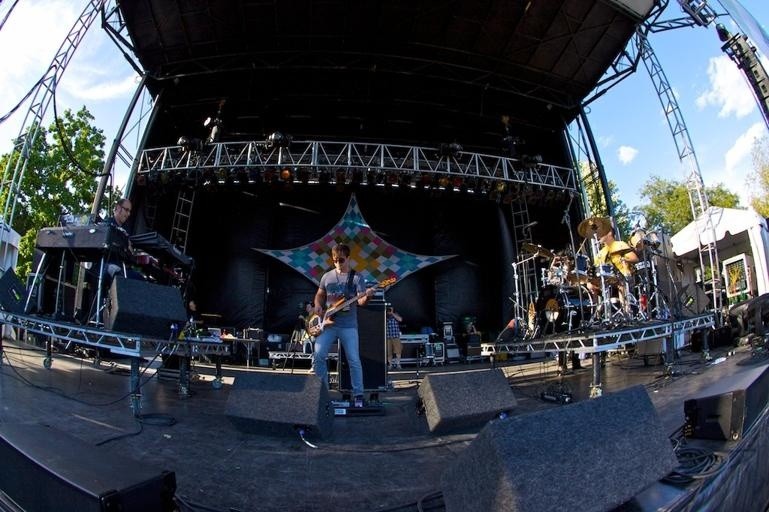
[693,326,733,349]
[104,275,189,339]
[416,368,519,436]
[439,384,679,512]
[672,283,711,319]
[684,364,769,440]
[223,373,335,441]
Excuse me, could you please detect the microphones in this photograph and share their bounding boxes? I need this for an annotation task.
[567,189,582,199]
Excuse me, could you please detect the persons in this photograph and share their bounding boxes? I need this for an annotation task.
[586,224,639,318]
[461,317,478,337]
[187,299,205,336]
[314,243,376,408]
[386,302,403,370]
[93,198,146,282]
[298,299,314,354]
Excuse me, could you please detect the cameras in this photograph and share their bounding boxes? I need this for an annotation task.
[387,307,392,312]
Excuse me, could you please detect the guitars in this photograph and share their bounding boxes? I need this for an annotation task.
[305,277,396,337]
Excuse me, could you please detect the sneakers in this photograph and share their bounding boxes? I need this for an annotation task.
[388,360,402,370]
[355,396,369,407]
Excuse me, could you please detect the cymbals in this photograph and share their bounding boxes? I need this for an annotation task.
[578,217,610,239]
[523,243,553,256]
[610,249,634,255]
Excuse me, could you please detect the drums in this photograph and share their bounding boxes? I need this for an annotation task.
[536,284,593,334]
[549,253,590,285]
[590,262,618,285]
[629,228,649,252]
[645,230,660,250]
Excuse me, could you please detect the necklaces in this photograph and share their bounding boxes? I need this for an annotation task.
[334,271,348,296]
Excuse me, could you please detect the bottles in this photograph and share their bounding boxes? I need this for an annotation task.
[169,322,179,342]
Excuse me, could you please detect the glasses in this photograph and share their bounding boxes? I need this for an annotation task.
[333,257,345,264]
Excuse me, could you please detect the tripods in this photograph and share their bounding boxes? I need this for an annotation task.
[501,199,670,342]
